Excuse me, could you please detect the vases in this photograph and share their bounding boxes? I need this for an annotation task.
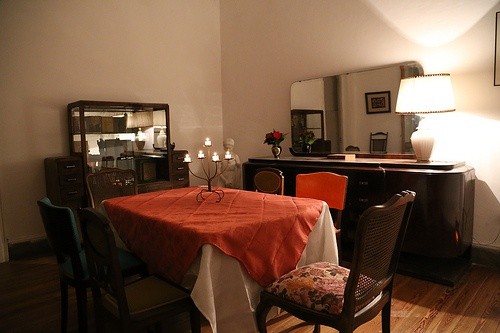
[271,145,282,157]
[303,144,312,152]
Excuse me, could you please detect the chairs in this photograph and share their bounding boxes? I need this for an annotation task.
[295,172,348,265]
[370,132,388,151]
[86,168,137,208]
[253,168,283,195]
[346,145,360,151]
[255,190,415,333]
[36,197,202,333]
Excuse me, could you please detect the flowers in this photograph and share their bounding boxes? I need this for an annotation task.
[300,129,314,139]
[266,130,284,143]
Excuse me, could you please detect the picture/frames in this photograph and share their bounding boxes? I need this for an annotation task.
[366,90,391,113]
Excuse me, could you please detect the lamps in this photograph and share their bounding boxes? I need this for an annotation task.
[395,75,458,162]
[182,138,232,202]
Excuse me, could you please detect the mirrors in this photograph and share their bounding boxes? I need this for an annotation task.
[291,110,324,153]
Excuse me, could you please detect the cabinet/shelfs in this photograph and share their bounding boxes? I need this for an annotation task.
[68,101,171,196]
[172,150,189,187]
[241,157,475,286]
